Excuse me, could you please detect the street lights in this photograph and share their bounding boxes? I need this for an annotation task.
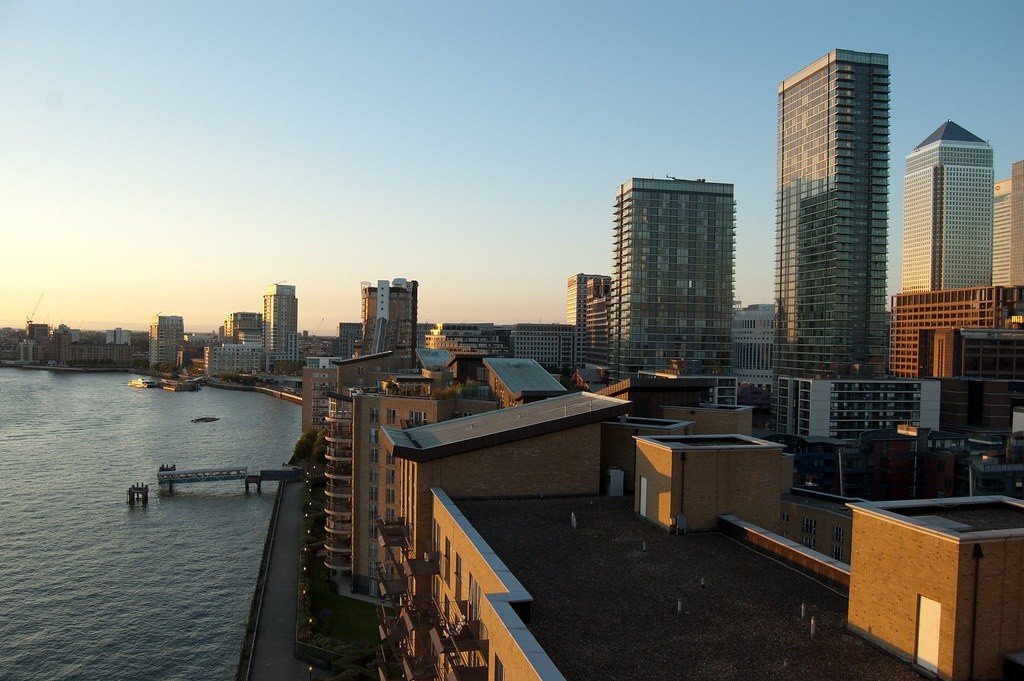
[300,465,317,681]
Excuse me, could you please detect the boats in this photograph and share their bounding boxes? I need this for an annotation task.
[128,378,157,388]
